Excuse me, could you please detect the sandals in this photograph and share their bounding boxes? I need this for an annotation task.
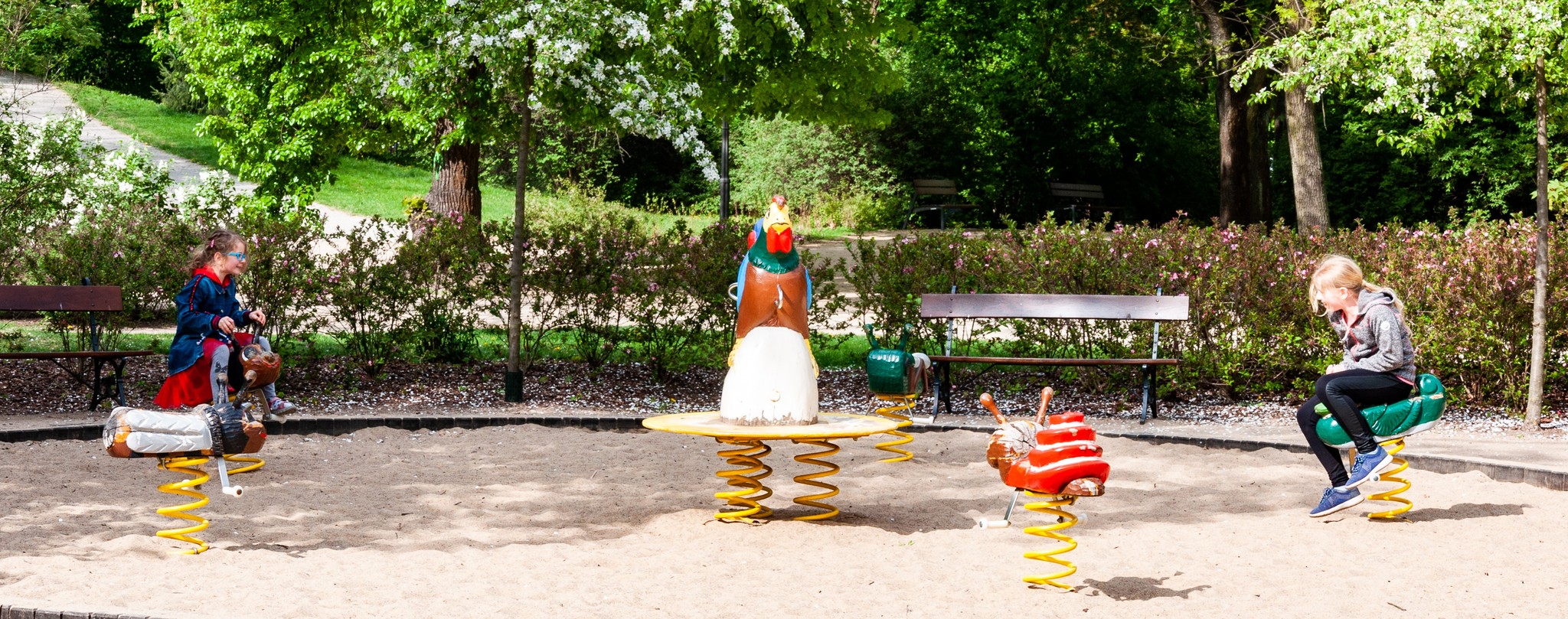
[268,397,296,415]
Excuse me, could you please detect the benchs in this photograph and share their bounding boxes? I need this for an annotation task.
[0,276,156,414]
[916,284,1192,425]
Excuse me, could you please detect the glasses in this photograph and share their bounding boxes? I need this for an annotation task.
[222,252,247,261]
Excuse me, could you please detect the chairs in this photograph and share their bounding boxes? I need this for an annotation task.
[1048,183,1123,228]
[901,179,979,232]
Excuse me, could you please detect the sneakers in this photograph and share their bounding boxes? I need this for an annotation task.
[1345,443,1394,492]
[1308,487,1364,517]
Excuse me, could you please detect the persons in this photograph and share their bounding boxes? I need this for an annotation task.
[1296,256,1416,517]
[153,229,297,416]
